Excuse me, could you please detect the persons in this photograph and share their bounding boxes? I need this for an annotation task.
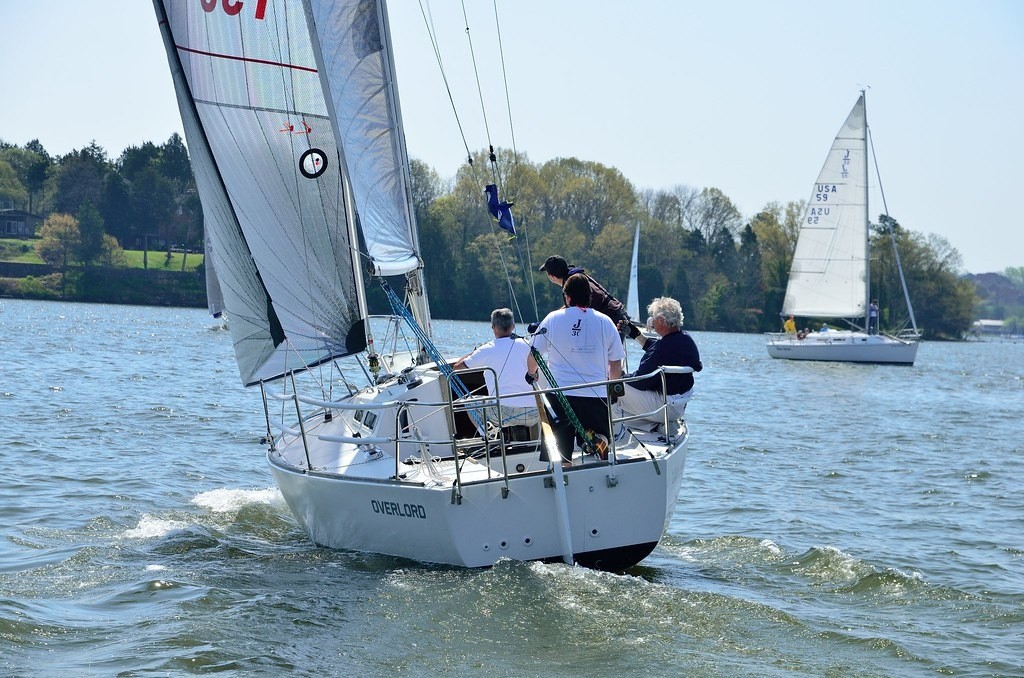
[784,316,797,340]
[525,273,626,466]
[797,323,830,341]
[538,255,631,377]
[452,308,539,439]
[868,299,879,336]
[611,296,700,443]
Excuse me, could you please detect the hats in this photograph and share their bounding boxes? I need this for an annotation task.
[539,255,576,273]
[790,315,794,318]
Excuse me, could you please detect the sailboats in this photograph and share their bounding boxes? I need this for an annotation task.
[766,88,922,369]
[152,0,691,571]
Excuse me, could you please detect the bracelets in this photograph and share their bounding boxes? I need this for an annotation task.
[528,367,538,377]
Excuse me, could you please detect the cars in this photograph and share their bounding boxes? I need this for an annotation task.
[170,243,199,254]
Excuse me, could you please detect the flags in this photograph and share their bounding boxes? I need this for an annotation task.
[485,183,515,234]
